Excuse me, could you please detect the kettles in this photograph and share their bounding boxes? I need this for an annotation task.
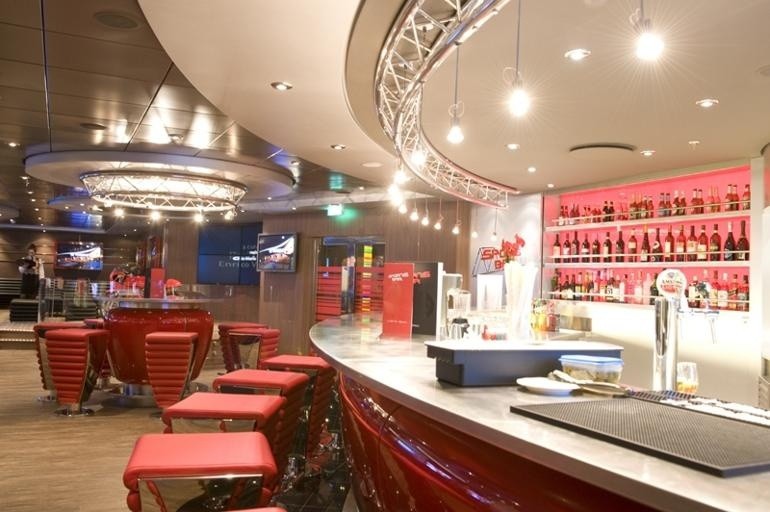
[449,318,470,340]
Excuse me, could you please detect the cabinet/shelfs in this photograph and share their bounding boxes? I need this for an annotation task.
[542,166,751,312]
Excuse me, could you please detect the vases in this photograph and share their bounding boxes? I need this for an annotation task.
[504,261,537,347]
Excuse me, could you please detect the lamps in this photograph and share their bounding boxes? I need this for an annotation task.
[79,171,248,225]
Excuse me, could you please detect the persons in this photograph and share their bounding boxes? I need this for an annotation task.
[18,243,45,300]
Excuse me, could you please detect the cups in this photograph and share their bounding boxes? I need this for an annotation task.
[676,360,700,395]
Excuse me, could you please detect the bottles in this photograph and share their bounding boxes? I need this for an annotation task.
[556,184,750,226]
[552,233,562,262]
[615,230,625,263]
[627,220,749,263]
[563,233,572,262]
[530,298,559,332]
[603,232,613,262]
[592,231,601,262]
[581,233,591,263]
[571,230,580,263]
[550,268,748,311]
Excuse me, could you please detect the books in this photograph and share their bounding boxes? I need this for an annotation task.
[413,259,444,336]
[375,260,414,336]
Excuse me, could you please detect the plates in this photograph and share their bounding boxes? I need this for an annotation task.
[515,376,581,395]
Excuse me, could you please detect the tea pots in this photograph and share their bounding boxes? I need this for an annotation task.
[446,288,471,318]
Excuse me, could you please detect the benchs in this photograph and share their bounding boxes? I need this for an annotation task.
[0,278,100,322]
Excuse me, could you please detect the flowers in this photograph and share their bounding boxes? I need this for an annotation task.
[500,234,526,263]
